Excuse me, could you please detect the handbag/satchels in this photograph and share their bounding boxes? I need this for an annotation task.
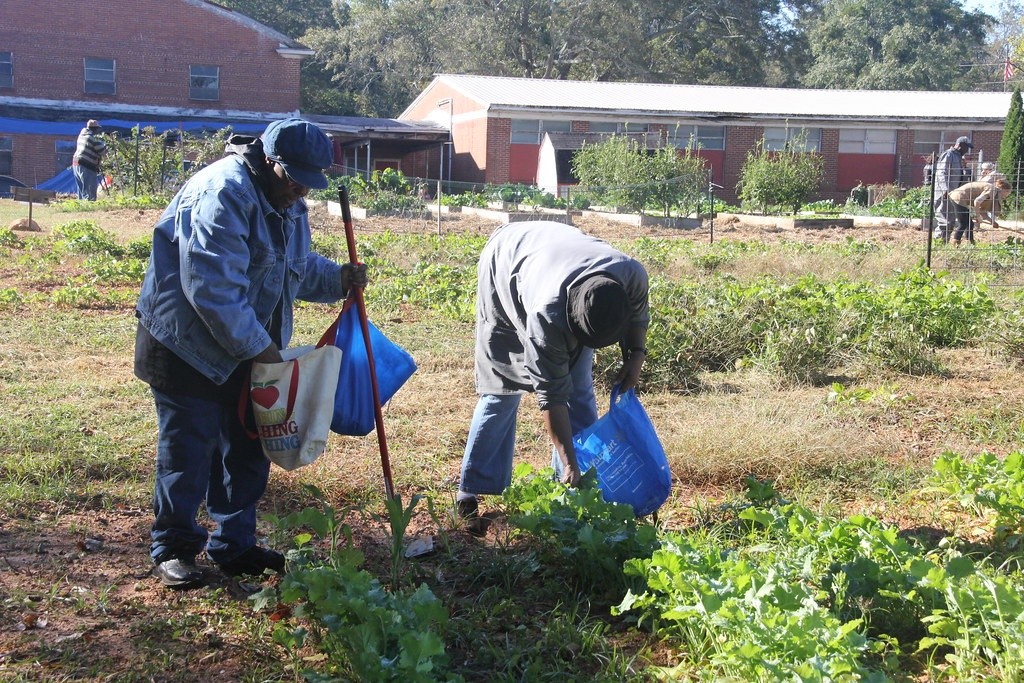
[332,300,418,437]
[557,382,671,519]
[250,266,366,472]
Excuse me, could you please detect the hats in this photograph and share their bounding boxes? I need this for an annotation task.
[262,119,333,189]
[956,135,974,148]
[566,271,633,349]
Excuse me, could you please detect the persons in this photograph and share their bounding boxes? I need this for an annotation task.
[134,118,369,586]
[454,220,651,538]
[72,119,108,201]
[924,136,1011,248]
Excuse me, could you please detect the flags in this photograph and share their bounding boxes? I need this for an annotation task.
[1005,58,1017,82]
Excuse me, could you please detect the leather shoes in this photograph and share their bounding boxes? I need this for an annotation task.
[215,545,285,575]
[454,494,481,533]
[151,543,207,586]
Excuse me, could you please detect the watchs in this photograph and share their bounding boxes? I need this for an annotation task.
[623,346,646,360]
